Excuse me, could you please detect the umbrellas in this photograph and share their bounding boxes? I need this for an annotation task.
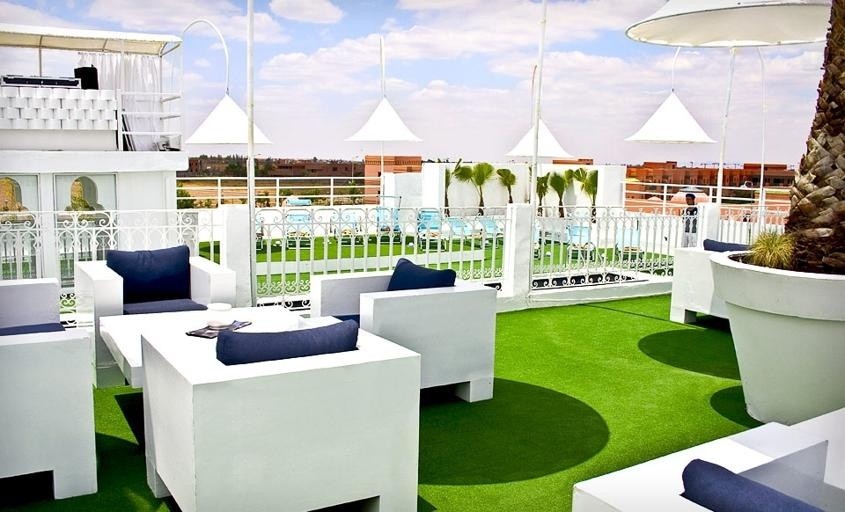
[185,93,272,145]
[345,96,423,147]
[624,0,839,50]
[623,91,720,146]
[508,118,575,161]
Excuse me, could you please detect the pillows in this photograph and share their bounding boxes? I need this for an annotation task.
[703,238,745,253]
[106,245,194,302]
[388,258,459,290]
[681,458,823,510]
[217,316,359,367]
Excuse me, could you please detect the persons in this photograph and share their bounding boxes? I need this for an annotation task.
[680,193,699,247]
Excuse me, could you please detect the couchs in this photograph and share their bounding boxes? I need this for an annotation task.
[310,261,498,403]
[668,243,734,324]
[569,422,843,511]
[1,277,96,506]
[74,248,239,367]
[138,312,421,512]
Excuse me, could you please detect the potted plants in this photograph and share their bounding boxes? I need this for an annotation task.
[709,0,844,423]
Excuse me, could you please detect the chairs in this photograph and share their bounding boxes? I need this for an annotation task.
[533,203,650,264]
[255,195,504,252]
